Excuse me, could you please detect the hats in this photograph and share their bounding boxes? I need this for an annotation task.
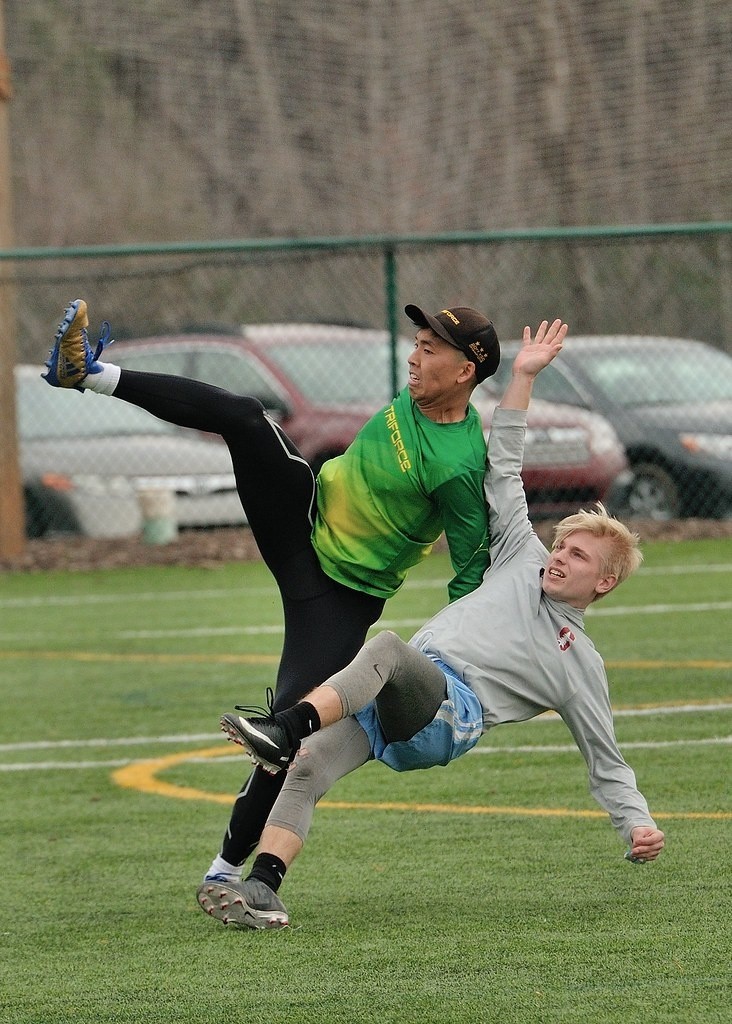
[404,303,501,384]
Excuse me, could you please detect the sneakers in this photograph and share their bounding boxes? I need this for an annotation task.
[39,298,115,394]
[219,687,292,775]
[196,872,288,930]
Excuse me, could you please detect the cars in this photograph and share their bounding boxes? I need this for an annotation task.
[106,323,629,523]
[14,365,248,537]
[495,335,732,525]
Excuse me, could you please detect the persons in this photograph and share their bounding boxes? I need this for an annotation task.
[41,299,501,886]
[195,318,666,930]
